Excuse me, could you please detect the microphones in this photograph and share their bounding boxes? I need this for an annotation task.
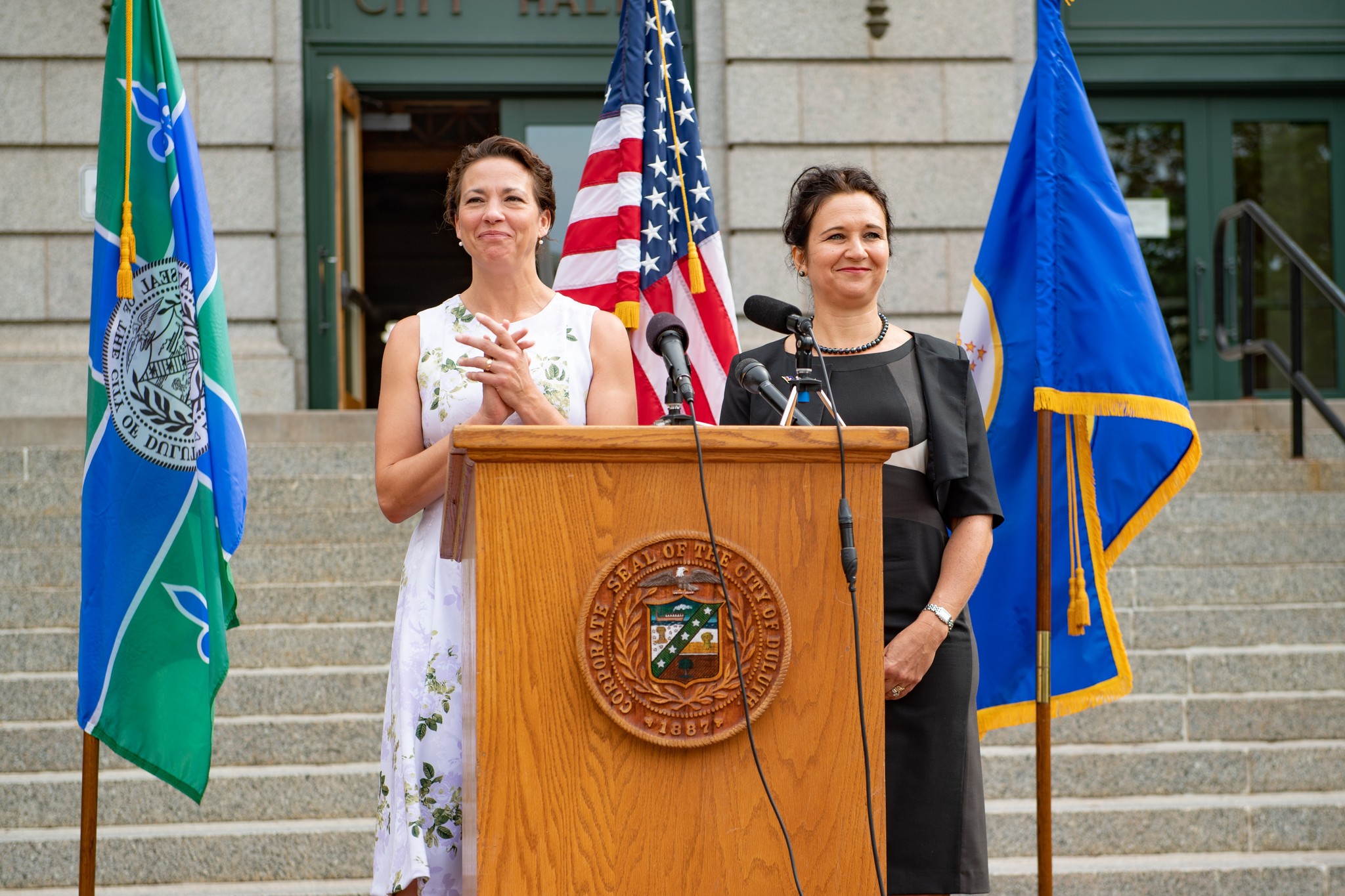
[735,358,814,425]
[744,295,811,335]
[646,312,695,402]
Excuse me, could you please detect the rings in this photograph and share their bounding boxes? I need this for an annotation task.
[891,685,905,697]
[485,358,492,371]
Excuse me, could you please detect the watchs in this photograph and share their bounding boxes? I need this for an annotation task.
[920,604,954,634]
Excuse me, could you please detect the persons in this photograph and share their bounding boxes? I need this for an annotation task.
[719,164,1006,896]
[367,135,639,896]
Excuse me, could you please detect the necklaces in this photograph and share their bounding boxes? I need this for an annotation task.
[809,312,888,354]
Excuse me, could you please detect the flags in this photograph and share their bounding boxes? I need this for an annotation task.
[74,0,249,805]
[554,0,742,427]
[954,0,1198,740]
